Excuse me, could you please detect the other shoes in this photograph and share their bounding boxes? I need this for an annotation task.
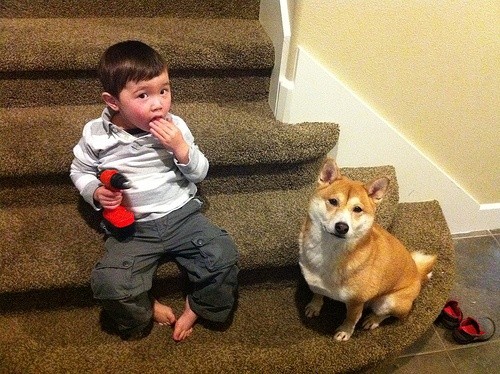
[439,299,463,329]
[452,317,495,344]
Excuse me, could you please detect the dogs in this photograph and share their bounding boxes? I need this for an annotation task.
[298,158,437,343]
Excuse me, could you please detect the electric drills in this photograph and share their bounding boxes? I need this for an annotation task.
[93,168,139,241]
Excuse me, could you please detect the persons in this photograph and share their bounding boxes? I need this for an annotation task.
[69,40,241,342]
